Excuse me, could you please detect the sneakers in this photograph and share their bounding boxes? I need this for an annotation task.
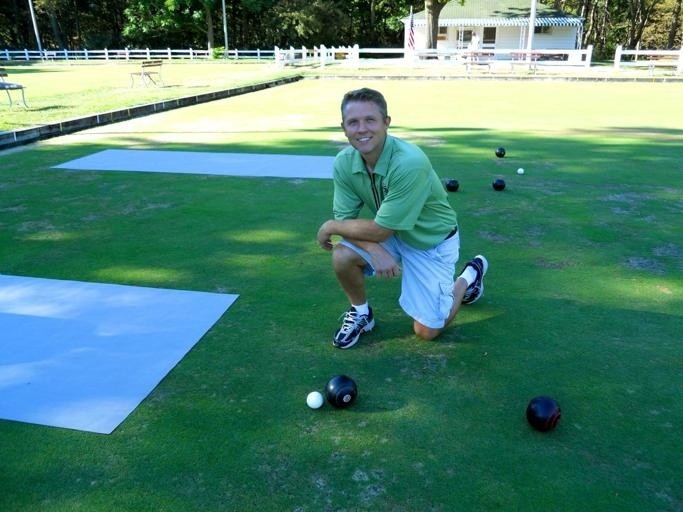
[333,305,375,350]
[457,255,489,305]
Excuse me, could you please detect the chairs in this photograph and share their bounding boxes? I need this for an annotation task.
[0,66,27,109]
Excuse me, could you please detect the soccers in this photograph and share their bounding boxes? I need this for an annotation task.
[493,180,505,191]
[446,179,458,191]
[496,148,505,158]
[327,374,357,409]
[526,396,562,432]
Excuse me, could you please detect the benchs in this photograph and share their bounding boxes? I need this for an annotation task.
[463,51,494,73]
[511,51,543,73]
[130,59,163,86]
[645,51,680,72]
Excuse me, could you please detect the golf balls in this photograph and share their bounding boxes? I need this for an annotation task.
[306,391,323,408]
[517,168,524,175]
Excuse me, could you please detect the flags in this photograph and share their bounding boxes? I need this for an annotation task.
[405,6,415,50]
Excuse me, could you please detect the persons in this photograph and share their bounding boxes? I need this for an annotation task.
[469,31,482,62]
[316,88,489,351]
